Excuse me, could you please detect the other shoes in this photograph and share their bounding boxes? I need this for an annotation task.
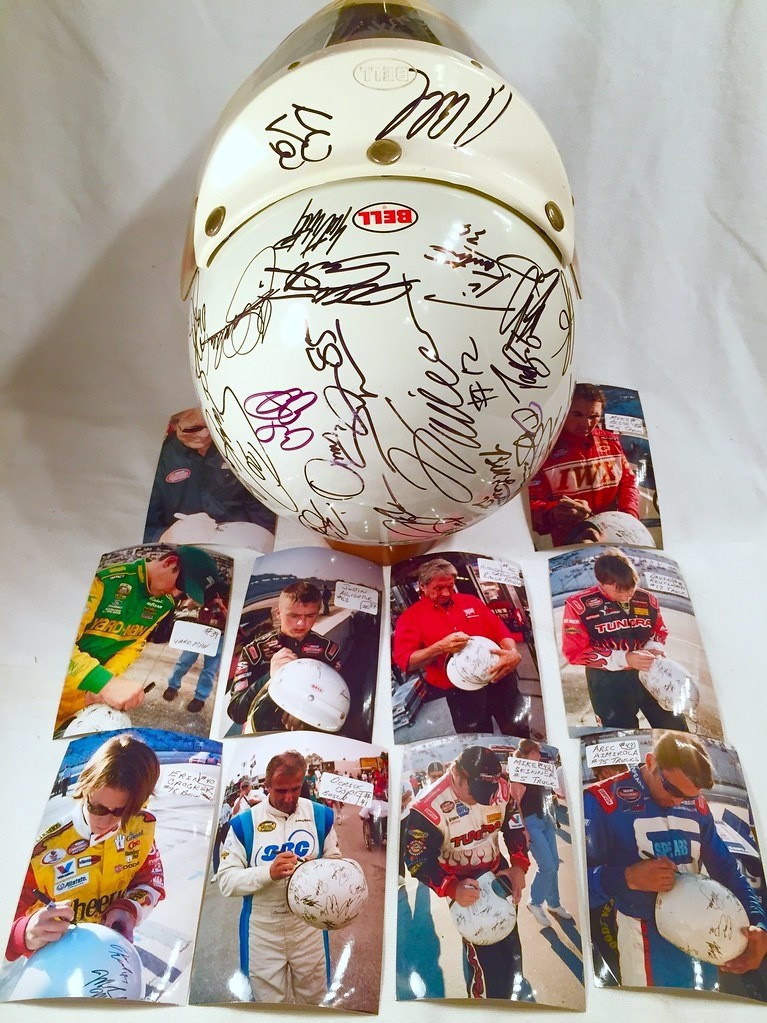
[546,905,572,919]
[526,902,551,927]
[162,687,177,701]
[211,874,219,883]
[188,698,204,712]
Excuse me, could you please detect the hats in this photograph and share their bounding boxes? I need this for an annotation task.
[175,545,220,606]
[457,745,503,805]
[241,781,250,788]
[374,787,385,799]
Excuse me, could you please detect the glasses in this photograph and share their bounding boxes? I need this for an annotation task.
[431,774,442,778]
[178,425,207,434]
[87,794,127,817]
[657,763,700,801]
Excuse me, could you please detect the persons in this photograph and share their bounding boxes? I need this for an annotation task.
[321,584,332,615]
[583,731,767,992]
[210,750,388,1008]
[528,383,640,548]
[392,559,530,741]
[402,738,572,1000]
[51,764,71,797]
[143,408,278,545]
[54,546,227,739]
[4,734,165,962]
[227,581,341,733]
[561,552,690,733]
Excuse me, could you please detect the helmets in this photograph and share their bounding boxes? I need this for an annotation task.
[268,658,351,732]
[638,655,700,722]
[654,871,750,965]
[449,871,517,945]
[286,858,368,930]
[446,636,502,691]
[573,511,657,547]
[181,0,583,543]
[8,923,146,1000]
